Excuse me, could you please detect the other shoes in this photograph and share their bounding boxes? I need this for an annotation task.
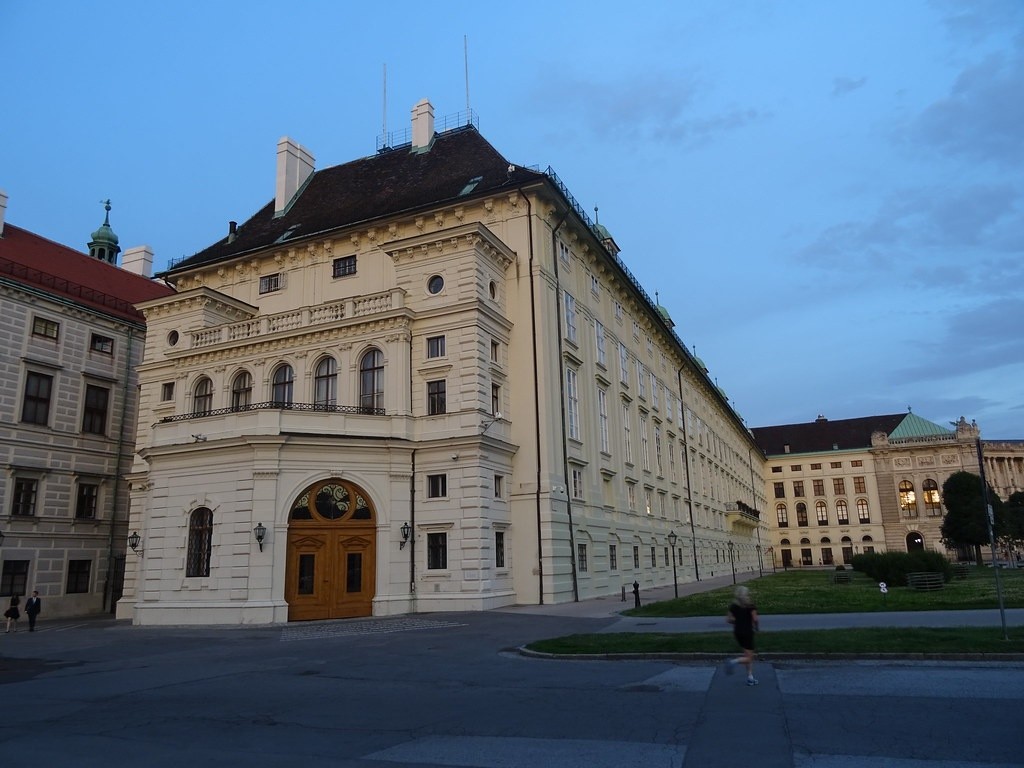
[747,678,759,686]
[725,658,735,676]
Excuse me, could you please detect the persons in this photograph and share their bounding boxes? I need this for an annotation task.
[4,592,21,633]
[725,586,760,685]
[959,416,977,432]
[24,591,41,632]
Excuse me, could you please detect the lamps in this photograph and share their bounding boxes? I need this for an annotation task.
[399,522,413,551]
[128,531,144,558]
[254,522,267,552]
